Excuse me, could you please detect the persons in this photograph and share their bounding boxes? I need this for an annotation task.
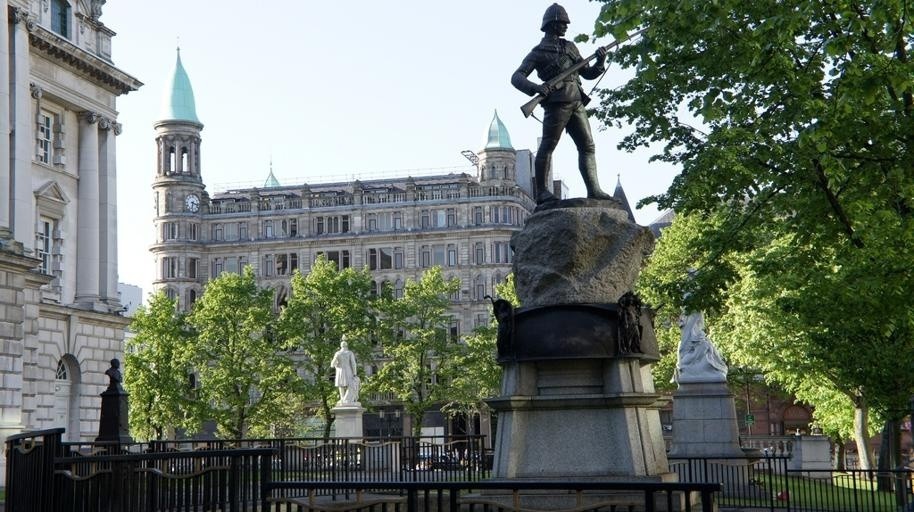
[104,359,123,384]
[331,342,357,399]
[511,3,613,204]
[678,268,705,354]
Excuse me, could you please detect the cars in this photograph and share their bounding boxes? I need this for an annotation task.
[418,453,462,471]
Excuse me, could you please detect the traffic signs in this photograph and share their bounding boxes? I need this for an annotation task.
[745,415,754,425]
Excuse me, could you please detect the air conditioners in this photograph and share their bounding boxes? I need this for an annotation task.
[663,425,672,431]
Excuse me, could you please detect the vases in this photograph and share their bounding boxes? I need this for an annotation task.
[741,448,764,460]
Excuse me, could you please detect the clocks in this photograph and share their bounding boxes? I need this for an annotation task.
[185,193,200,213]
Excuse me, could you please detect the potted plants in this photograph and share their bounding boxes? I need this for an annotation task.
[814,422,822,434]
